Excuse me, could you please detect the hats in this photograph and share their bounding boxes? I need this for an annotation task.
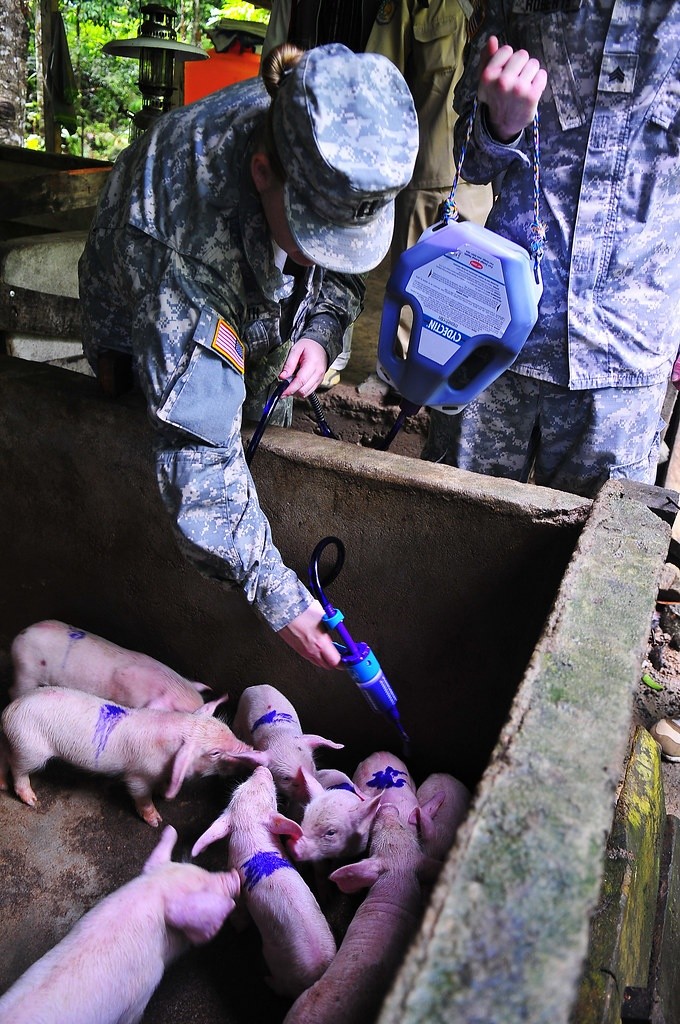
[271,44,419,274]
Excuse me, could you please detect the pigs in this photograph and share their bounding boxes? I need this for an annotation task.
[1,618,472,1024]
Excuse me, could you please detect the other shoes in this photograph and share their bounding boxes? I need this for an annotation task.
[651,714,680,762]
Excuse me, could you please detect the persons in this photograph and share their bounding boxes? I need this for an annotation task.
[79,43,421,674]
[456,0,679,489]
[260,1,467,400]
